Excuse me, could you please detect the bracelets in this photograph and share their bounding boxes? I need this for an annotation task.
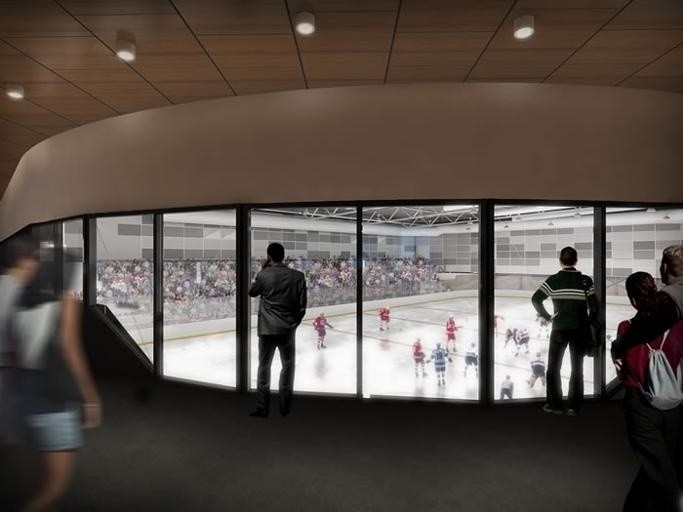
[79,401,102,408]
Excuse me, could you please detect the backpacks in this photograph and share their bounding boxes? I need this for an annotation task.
[626,318,683,411]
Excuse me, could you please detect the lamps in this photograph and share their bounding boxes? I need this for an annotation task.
[293,12,317,37]
[115,39,138,61]
[510,14,538,40]
[5,82,28,102]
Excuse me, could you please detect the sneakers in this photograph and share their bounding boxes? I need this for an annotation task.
[542,403,576,416]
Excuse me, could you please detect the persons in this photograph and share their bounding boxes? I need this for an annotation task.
[412,314,479,387]
[249,242,307,417]
[312,310,335,350]
[495,312,550,359]
[500,373,514,399]
[379,306,390,331]
[529,351,546,387]
[97,254,452,326]
[531,246,600,416]
[611,244,683,512]
[8,254,102,512]
[0,237,42,512]
